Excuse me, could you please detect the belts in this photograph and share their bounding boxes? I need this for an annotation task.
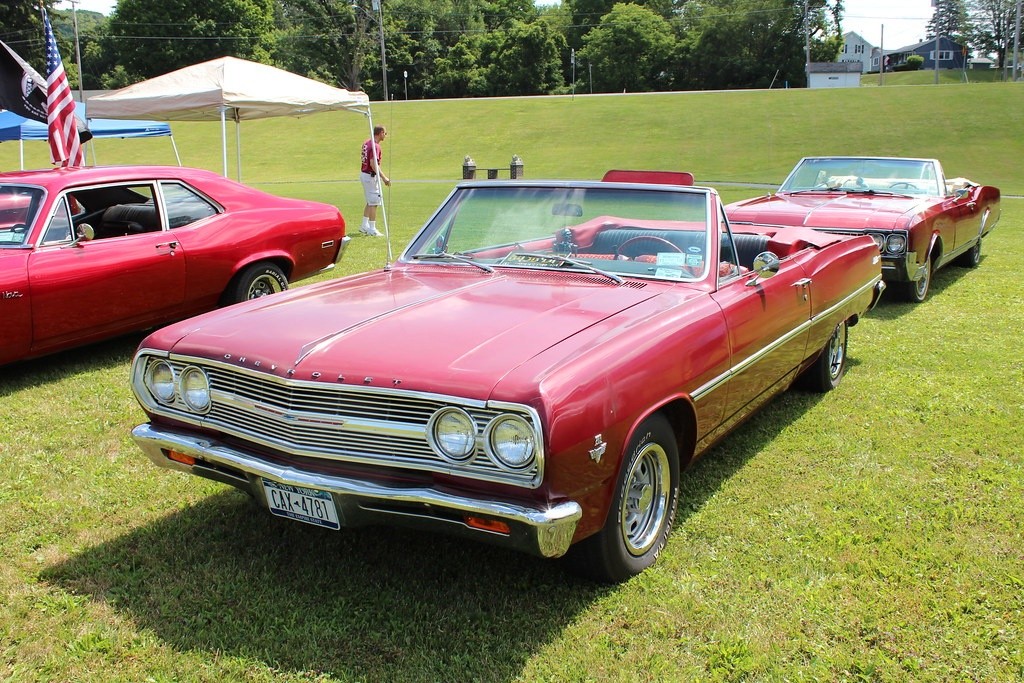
[362,171,377,175]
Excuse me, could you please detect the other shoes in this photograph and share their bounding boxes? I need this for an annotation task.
[359,226,367,233]
[366,229,383,237]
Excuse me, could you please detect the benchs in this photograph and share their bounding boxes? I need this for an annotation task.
[102,205,159,230]
[461,153,523,179]
[591,229,770,273]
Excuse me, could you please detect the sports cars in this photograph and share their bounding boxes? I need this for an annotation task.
[2,162,350,373]
[128,169,886,586]
[715,154,1002,302]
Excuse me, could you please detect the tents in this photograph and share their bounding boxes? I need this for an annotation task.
[85,56,392,263]
[0,100,181,171]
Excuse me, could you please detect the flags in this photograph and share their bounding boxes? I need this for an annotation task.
[40,0,85,168]
[0,39,94,144]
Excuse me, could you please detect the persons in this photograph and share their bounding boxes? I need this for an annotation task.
[359,125,391,237]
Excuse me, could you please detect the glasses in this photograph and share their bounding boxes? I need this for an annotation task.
[382,131,387,136]
